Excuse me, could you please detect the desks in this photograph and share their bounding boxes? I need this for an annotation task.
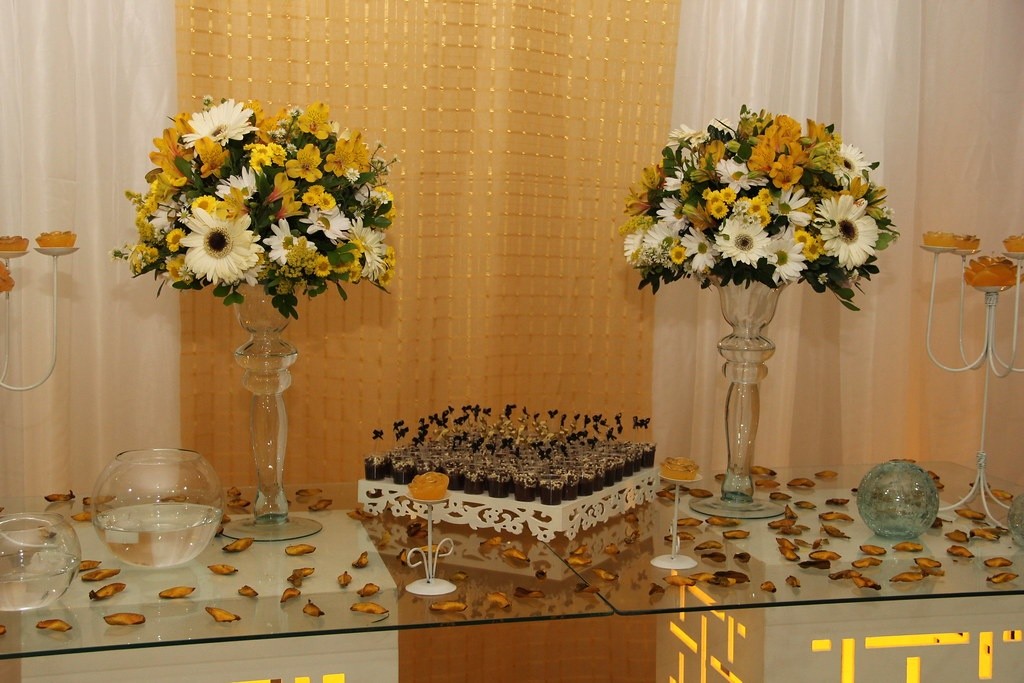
[1,461,1022,659]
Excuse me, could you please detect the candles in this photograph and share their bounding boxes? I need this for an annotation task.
[963,256,1024,287]
[0,235,29,251]
[408,471,449,500]
[661,457,698,479]
[954,234,980,250]
[1003,233,1024,252]
[35,230,77,248]
[0,261,15,292]
[923,230,954,247]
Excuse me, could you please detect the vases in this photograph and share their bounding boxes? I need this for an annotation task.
[221,282,324,542]
[689,269,794,519]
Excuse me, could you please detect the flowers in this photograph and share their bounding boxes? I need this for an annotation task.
[109,94,397,320]
[620,104,901,311]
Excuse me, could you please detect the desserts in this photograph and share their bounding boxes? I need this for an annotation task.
[364,424,655,506]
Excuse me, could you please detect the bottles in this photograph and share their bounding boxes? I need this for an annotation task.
[90,448,225,570]
[0,510,82,612]
